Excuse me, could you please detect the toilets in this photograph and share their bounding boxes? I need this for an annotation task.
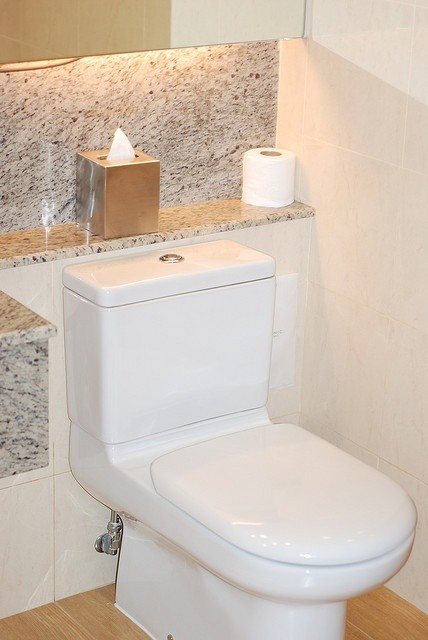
[63,239,417,640]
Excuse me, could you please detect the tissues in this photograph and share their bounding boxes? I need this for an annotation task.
[76,129,160,239]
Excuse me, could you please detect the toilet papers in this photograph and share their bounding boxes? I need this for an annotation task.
[241,148,296,208]
